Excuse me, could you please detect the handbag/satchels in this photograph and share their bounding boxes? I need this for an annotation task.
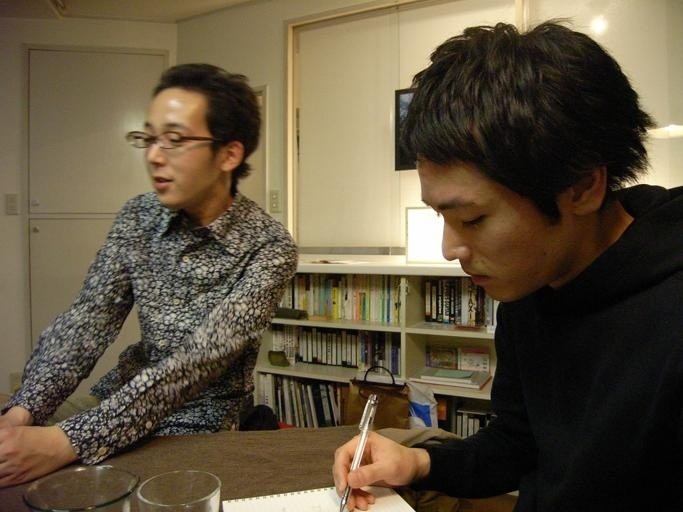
[345,367,410,430]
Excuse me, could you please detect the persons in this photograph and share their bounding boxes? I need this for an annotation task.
[332,17,683,512]
[1,63,299,489]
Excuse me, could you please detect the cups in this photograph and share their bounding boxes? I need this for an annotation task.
[20,464,139,512]
[136,469,223,512]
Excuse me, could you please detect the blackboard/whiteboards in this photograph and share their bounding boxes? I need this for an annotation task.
[406,207,461,264]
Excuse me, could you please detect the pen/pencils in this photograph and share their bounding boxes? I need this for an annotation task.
[340,393,379,512]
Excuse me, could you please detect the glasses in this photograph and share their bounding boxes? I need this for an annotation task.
[126,131,212,150]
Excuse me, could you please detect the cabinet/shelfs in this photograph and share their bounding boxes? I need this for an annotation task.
[252,262,500,439]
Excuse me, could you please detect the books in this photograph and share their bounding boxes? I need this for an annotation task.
[222,485,416,512]
[259,274,400,428]
[423,278,501,438]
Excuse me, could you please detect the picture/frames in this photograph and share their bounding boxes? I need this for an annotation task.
[394,88,417,171]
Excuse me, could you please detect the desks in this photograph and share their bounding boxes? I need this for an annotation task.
[1,423,518,511]
